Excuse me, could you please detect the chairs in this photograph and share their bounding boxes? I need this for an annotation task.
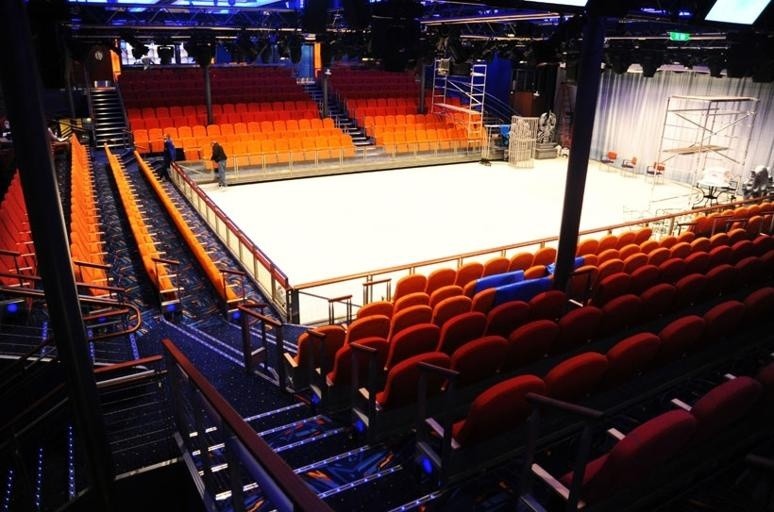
[1,118,256,318]
[644,162,665,184]
[621,157,638,177]
[114,58,489,167]
[296,200,774,511]
[599,151,617,171]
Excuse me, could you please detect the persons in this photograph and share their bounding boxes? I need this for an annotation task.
[164,134,175,162]
[209,140,227,186]
